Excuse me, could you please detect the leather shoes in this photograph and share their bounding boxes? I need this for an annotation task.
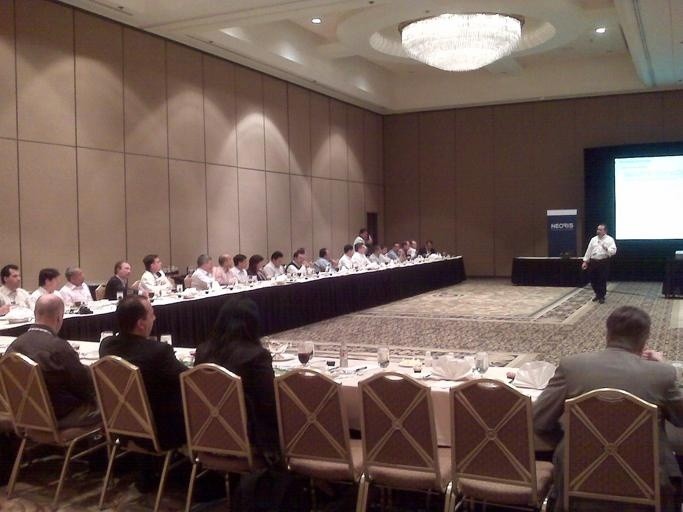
[599,298,605,305]
[592,296,598,302]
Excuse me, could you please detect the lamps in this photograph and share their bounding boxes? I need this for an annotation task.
[398,12,525,73]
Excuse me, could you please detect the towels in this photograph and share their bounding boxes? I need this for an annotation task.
[428,355,475,381]
[513,360,556,390]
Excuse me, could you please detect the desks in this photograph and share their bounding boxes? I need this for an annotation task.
[662,257,683,299]
[1,336,683,461]
[0,255,467,348]
[511,256,594,288]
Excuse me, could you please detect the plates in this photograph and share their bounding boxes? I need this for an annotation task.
[85,356,99,359]
[399,361,416,367]
[272,355,294,361]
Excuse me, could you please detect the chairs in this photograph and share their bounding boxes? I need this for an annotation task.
[166,276,177,292]
[0,353,106,510]
[562,389,661,511]
[183,273,192,288]
[179,361,279,511]
[88,355,188,512]
[273,368,387,512]
[448,378,558,511]
[94,281,107,301]
[360,372,469,512]
[131,277,140,294]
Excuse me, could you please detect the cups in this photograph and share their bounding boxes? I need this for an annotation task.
[100,331,112,342]
[377,346,389,370]
[160,334,172,346]
[75,298,81,306]
[298,343,309,369]
[304,341,314,360]
[475,352,488,377]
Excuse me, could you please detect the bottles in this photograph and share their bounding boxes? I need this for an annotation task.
[339,341,348,369]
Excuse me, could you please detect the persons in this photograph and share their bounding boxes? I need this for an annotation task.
[3,294,101,428]
[0,265,31,317]
[285,247,314,275]
[192,294,279,452]
[190,253,216,291]
[312,247,339,274]
[337,244,355,270]
[105,260,132,300]
[398,241,410,260]
[379,246,391,263]
[59,265,94,306]
[581,223,618,304]
[387,241,400,260]
[246,254,272,281]
[138,254,173,297]
[530,305,683,512]
[230,254,248,284]
[351,242,372,268]
[353,228,373,251]
[264,251,285,277]
[418,240,437,265]
[407,240,417,260]
[370,245,382,265]
[98,294,188,448]
[30,268,66,315]
[212,253,238,286]
